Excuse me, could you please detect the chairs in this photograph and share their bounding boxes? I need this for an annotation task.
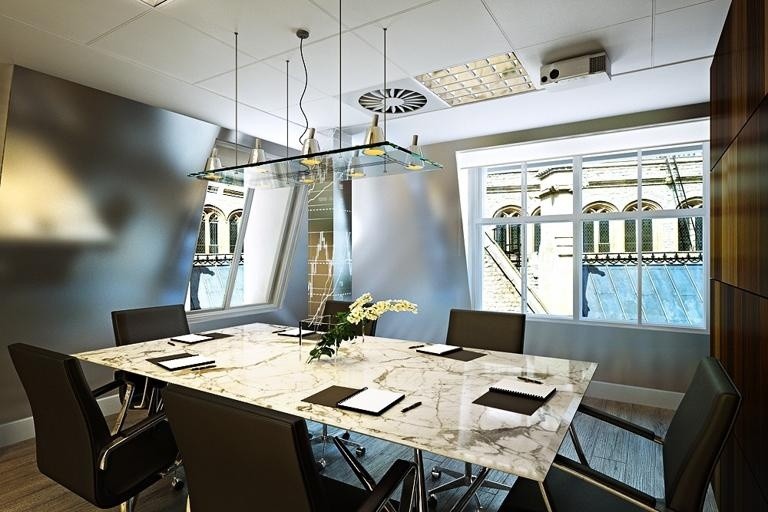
[109,304,209,413]
[185,401,418,511]
[296,300,380,459]
[426,308,525,508]
[8,341,194,508]
[502,352,743,510]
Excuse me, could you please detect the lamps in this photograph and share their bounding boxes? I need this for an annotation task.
[188,28,444,187]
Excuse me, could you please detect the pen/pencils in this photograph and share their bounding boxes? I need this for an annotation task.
[409,345,424,349]
[168,342,175,346]
[401,402,421,412]
[272,330,286,333]
[517,377,542,384]
[191,366,216,370]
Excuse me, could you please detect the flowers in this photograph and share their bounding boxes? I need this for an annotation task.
[306,293,418,363]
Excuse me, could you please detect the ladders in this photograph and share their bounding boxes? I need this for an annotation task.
[666,156,700,251]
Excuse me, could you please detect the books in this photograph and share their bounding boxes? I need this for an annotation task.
[145,353,217,371]
[472,372,558,417]
[415,343,487,362]
[278,327,325,341]
[300,385,405,415]
[170,331,234,345]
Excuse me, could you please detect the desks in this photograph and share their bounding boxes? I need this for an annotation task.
[66,321,601,479]
